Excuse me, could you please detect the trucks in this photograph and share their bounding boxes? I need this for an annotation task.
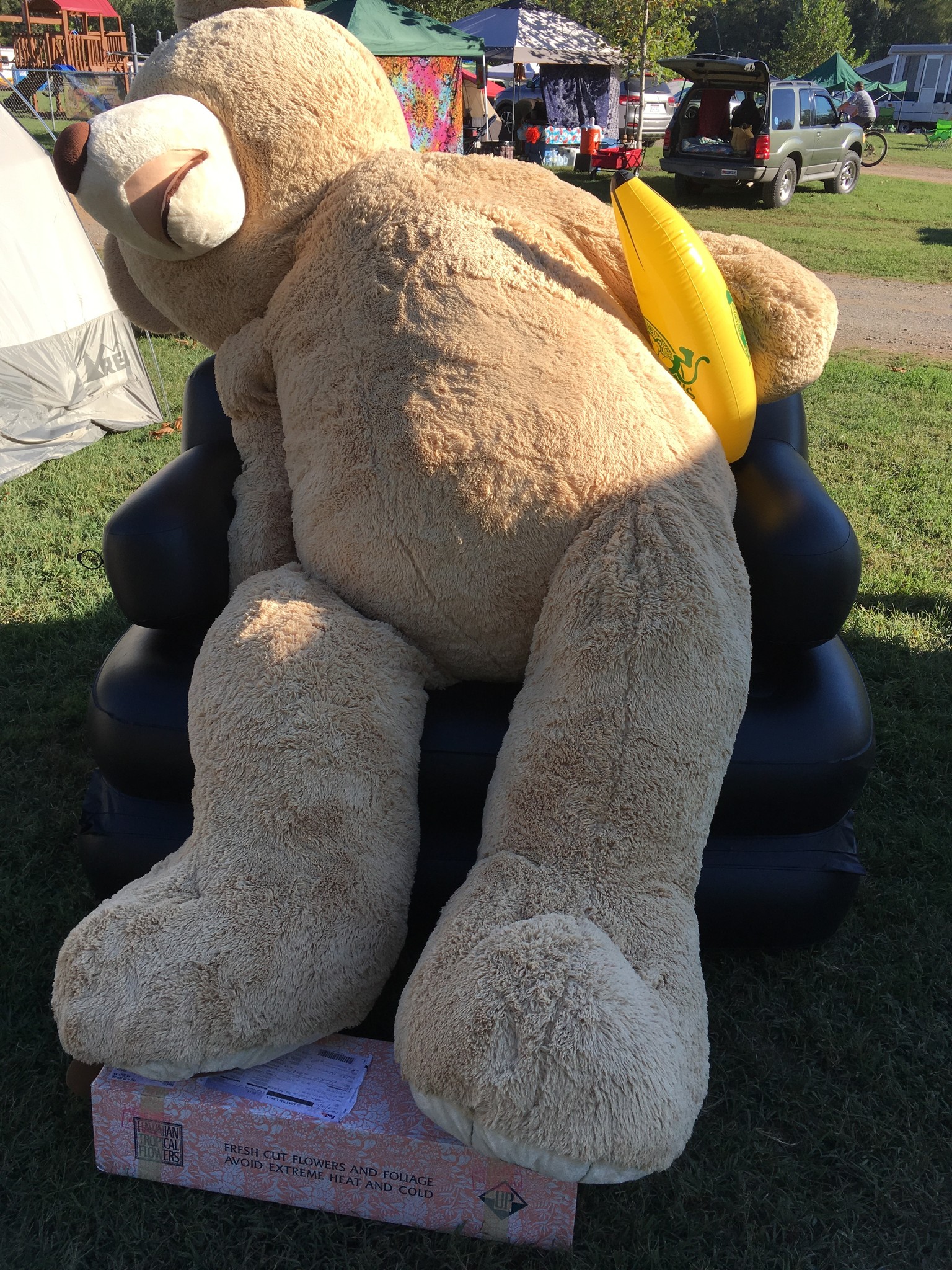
[855,44,952,133]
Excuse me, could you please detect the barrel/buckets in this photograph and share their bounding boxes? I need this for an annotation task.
[580,124,601,155]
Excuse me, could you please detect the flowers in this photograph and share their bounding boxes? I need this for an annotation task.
[524,126,541,146]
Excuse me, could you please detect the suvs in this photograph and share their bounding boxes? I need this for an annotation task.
[495,72,761,147]
[655,53,866,209]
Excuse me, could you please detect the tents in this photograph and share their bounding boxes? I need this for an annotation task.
[1,105,161,483]
[304,0,631,154]
[783,51,908,134]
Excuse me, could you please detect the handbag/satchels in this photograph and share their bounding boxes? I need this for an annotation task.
[731,123,754,151]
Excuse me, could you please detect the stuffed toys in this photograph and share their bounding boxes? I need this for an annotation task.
[48,0,842,1185]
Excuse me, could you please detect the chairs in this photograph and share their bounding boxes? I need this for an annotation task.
[464,114,498,155]
[731,97,761,133]
[873,106,896,133]
[922,118,952,151]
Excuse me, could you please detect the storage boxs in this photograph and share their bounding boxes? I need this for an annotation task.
[523,138,642,173]
[88,1032,580,1255]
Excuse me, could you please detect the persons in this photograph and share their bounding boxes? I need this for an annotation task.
[838,81,877,126]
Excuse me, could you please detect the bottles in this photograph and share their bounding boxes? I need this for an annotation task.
[557,154,563,166]
[544,153,552,167]
[550,150,557,166]
[562,154,568,166]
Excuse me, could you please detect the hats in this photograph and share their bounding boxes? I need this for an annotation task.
[854,81,863,87]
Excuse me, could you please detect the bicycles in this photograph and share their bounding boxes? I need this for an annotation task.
[839,111,888,167]
